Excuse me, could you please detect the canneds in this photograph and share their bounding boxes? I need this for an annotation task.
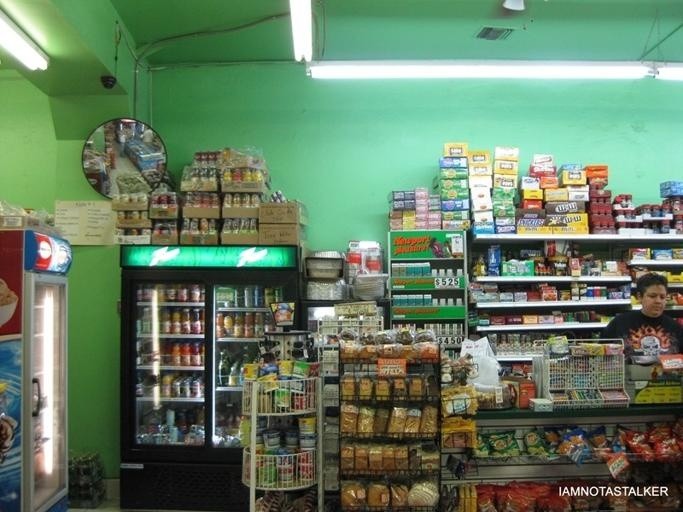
[135,279,208,302]
[173,375,205,397]
[235,283,283,307]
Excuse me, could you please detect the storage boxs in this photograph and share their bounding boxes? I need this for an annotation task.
[304,255,388,300]
[256,201,308,246]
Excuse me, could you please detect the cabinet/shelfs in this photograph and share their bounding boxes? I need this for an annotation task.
[240,377,324,511]
[389,233,683,360]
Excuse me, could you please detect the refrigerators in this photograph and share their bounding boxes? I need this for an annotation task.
[0,229,72,511]
[119,242,307,511]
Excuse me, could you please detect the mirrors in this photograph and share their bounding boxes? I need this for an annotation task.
[82,118,168,202]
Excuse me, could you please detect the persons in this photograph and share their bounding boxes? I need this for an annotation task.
[595,271,682,362]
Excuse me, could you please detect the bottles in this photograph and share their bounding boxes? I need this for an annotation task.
[137,283,204,435]
[112,147,287,238]
[218,344,260,386]
[215,312,283,338]
